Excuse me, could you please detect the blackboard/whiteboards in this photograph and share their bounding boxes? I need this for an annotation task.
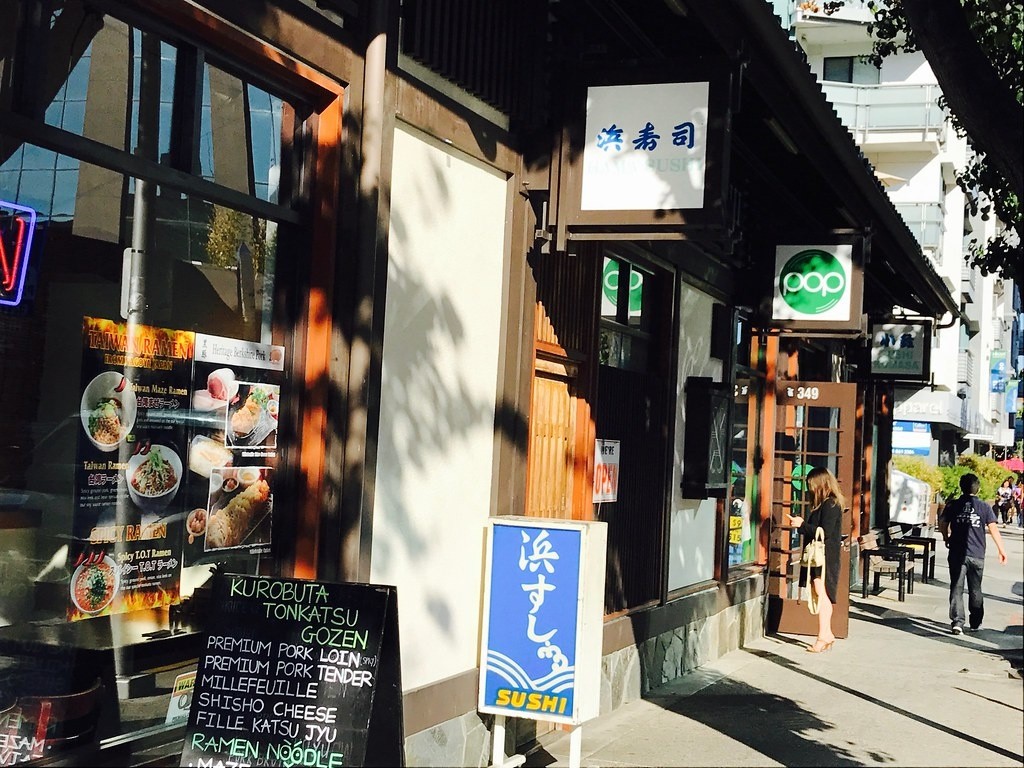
[179,571,409,767]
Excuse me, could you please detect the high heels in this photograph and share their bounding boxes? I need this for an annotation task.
[807,639,835,653]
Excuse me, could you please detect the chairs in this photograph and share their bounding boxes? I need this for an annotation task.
[884,524,936,585]
[857,533,915,603]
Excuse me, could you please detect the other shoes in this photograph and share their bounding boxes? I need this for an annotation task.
[970,626,980,631]
[952,626,963,636]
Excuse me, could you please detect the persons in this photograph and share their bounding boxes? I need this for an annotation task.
[938,473,1005,633]
[992,480,1013,527]
[1006,476,1024,527]
[784,467,846,654]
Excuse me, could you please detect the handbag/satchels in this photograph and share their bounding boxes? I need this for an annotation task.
[800,527,825,568]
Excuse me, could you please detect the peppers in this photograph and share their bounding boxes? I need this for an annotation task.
[74,548,104,566]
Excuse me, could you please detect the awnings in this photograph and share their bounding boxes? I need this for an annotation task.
[549,0,958,358]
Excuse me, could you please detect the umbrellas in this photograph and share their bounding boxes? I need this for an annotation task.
[999,458,1024,471]
